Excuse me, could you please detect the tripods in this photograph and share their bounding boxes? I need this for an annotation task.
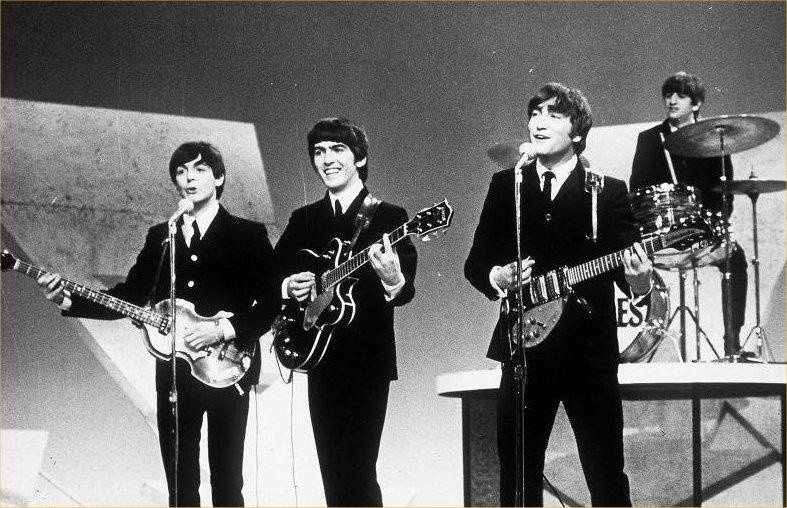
[735,197,785,366]
[642,275,724,362]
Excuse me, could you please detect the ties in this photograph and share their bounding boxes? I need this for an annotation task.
[542,170,555,201]
[334,198,344,220]
[190,219,202,248]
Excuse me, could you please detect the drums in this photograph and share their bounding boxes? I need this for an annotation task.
[629,182,737,271]
[613,268,670,364]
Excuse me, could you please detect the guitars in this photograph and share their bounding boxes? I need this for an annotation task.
[0,249,257,390]
[500,211,726,350]
[269,198,454,371]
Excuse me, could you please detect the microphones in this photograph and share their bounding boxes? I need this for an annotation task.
[166,197,196,234]
[513,141,539,180]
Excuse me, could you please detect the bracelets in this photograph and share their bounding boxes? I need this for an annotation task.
[214,318,222,342]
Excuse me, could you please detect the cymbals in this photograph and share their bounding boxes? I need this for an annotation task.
[710,177,787,194]
[661,114,780,160]
[488,140,589,169]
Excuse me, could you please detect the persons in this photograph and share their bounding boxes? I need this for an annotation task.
[272,117,417,508]
[464,81,652,508]
[38,142,279,507]
[630,71,755,358]
